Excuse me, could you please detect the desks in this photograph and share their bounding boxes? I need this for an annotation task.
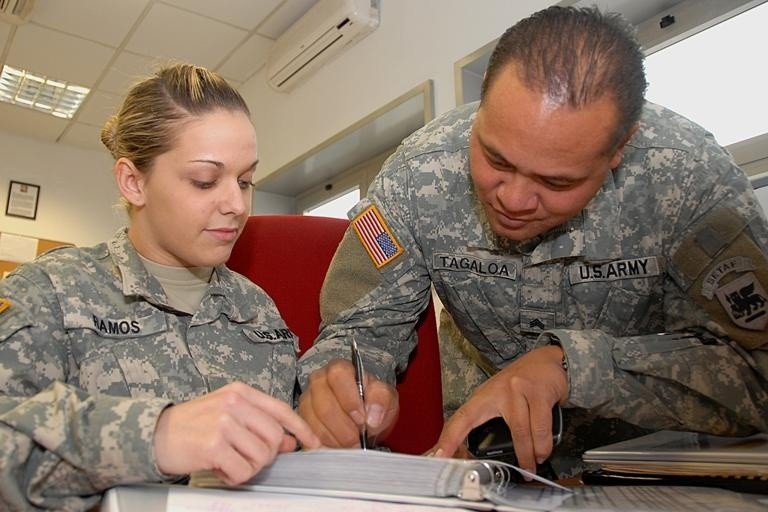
[87,474,765,510]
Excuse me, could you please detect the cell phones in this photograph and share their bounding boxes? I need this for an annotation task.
[473,423,517,464]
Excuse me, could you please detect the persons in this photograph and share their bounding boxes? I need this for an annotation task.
[296,3,767,481]
[1,63,329,510]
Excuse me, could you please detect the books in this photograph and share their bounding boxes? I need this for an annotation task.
[175,425,575,511]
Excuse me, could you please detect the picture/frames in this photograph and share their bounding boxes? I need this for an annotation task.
[4,178,43,220]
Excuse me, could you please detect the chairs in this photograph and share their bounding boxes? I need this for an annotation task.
[223,213,454,456]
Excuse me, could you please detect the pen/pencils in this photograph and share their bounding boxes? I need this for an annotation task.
[351,337,368,452]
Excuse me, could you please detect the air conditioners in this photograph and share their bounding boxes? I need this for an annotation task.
[263,0,382,96]
